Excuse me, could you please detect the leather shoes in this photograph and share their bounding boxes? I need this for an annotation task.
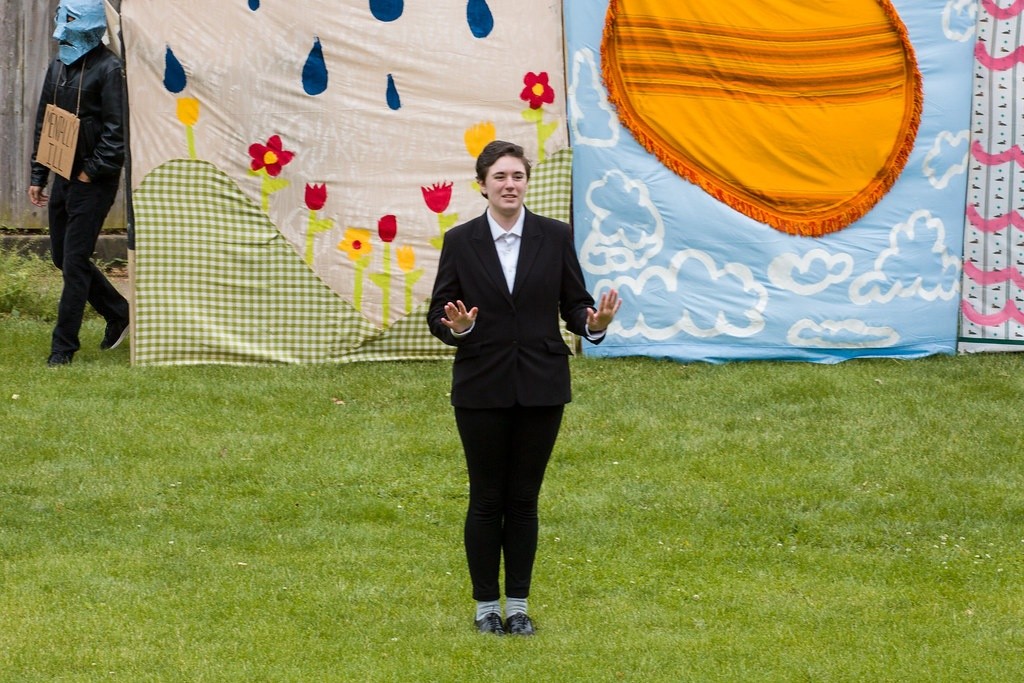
[474,612,533,639]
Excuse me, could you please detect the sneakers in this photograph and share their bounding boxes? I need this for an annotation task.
[101,304,130,351]
[47,343,75,369]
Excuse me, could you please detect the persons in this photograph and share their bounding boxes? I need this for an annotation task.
[426,140,622,636]
[29,0,129,368]
